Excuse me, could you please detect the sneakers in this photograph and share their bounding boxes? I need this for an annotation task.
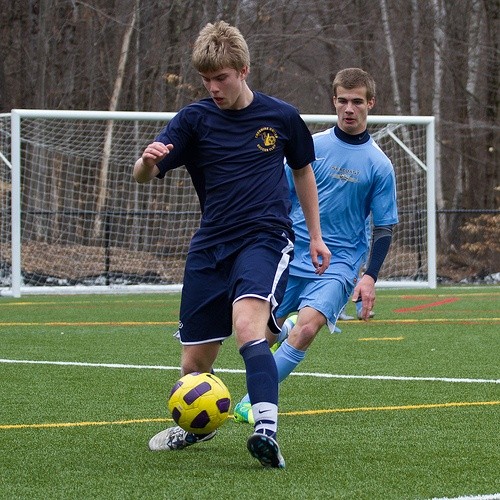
[270,315,298,356]
[148,425,218,451]
[247,433,286,468]
[232,401,254,426]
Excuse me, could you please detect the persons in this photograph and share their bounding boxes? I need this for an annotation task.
[233,68,400,423]
[132,20,332,468]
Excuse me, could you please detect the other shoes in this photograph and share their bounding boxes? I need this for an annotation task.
[338,312,354,321]
[356,308,374,320]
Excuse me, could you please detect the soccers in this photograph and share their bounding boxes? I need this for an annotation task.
[165,371,232,431]
